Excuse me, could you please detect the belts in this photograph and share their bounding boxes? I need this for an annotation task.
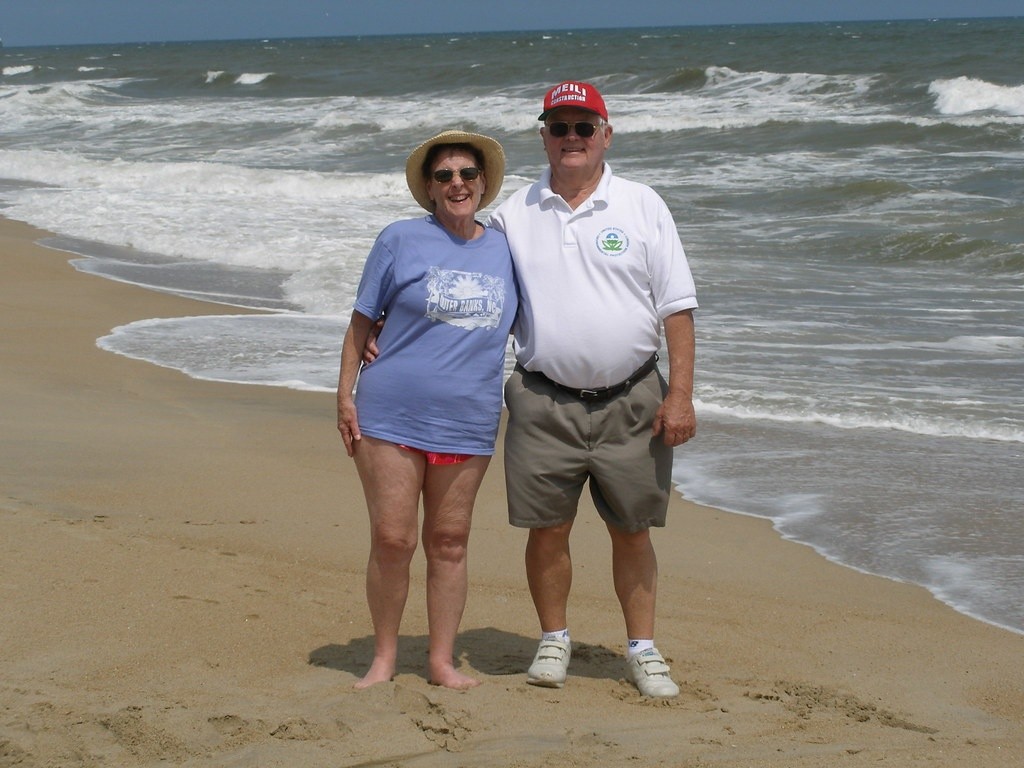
[518,353,655,402]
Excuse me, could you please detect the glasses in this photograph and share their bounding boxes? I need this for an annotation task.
[545,122,601,137]
[430,167,483,183]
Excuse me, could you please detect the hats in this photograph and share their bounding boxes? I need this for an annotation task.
[538,80,608,123]
[406,131,506,212]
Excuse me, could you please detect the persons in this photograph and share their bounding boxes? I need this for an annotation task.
[336,132,521,690]
[481,79,700,697]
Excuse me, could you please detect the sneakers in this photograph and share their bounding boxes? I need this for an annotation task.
[526,635,572,688]
[624,648,679,699]
